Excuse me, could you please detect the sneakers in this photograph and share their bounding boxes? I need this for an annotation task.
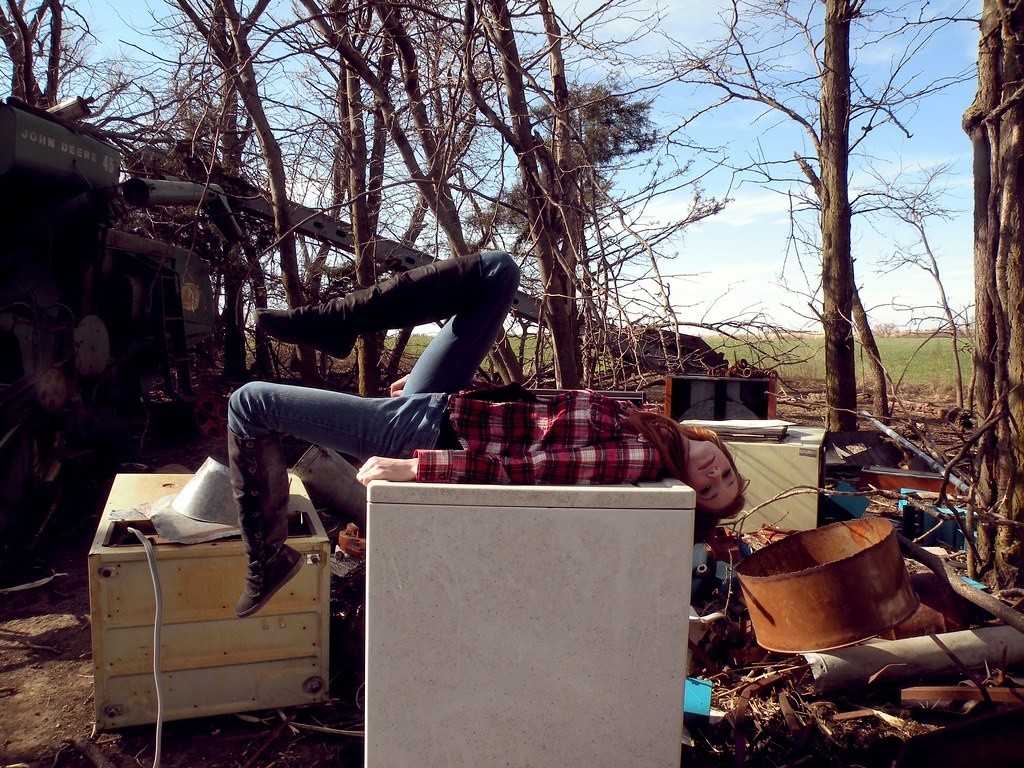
[235,545,305,618]
[253,306,357,359]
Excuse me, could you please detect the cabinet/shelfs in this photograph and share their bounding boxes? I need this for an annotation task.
[663,374,776,421]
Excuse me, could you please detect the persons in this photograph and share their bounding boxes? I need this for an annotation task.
[226,248,747,618]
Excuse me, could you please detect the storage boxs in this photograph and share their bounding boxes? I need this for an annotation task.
[721,425,826,536]
[88,473,332,732]
